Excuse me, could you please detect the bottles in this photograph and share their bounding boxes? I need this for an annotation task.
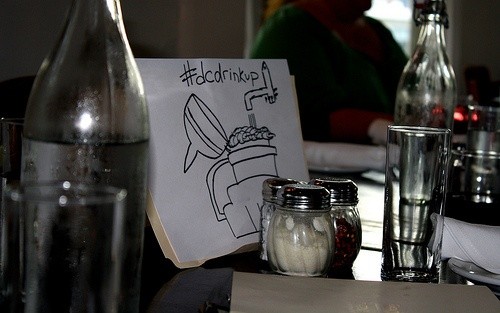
[14,0,151,313]
[261,174,364,281]
[393,0,458,125]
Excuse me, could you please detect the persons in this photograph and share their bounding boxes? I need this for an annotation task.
[245,0,411,146]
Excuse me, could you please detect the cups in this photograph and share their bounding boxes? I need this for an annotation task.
[452,104,500,204]
[383,126,451,282]
[0,115,128,312]
